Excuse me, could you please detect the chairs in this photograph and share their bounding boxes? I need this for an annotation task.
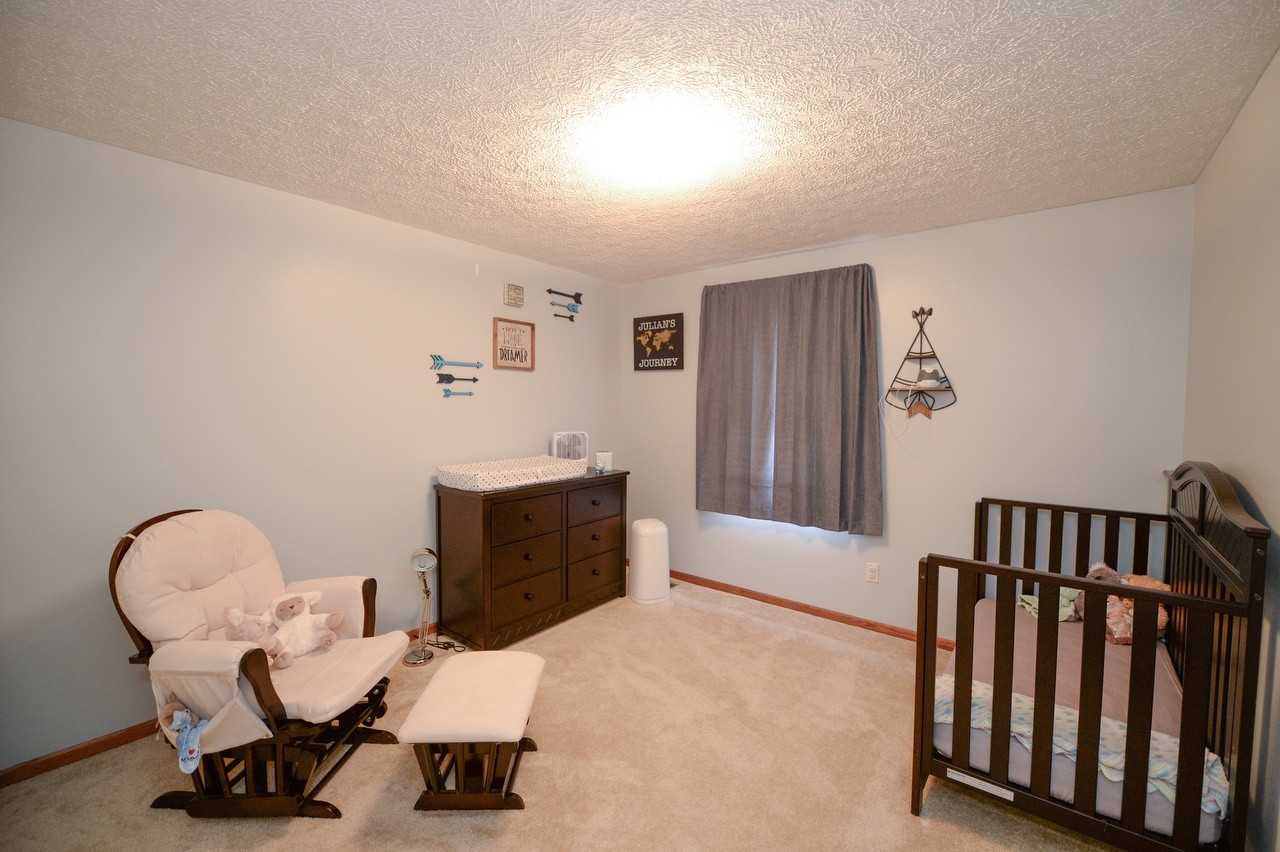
[109,509,411,817]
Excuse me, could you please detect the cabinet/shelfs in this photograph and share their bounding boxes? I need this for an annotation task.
[434,467,629,651]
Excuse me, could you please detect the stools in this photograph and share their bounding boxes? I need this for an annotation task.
[396,651,545,809]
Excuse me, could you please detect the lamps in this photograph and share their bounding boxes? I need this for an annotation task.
[403,548,438,666]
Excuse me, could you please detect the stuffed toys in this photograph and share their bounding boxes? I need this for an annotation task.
[258,591,344,670]
[1075,562,1172,645]
[225,605,281,669]
[160,691,198,732]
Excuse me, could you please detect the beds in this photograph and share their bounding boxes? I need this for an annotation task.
[911,461,1270,852]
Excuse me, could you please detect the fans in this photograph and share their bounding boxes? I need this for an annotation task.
[550,431,588,461]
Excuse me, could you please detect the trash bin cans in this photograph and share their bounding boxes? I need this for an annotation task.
[628,519,670,604]
